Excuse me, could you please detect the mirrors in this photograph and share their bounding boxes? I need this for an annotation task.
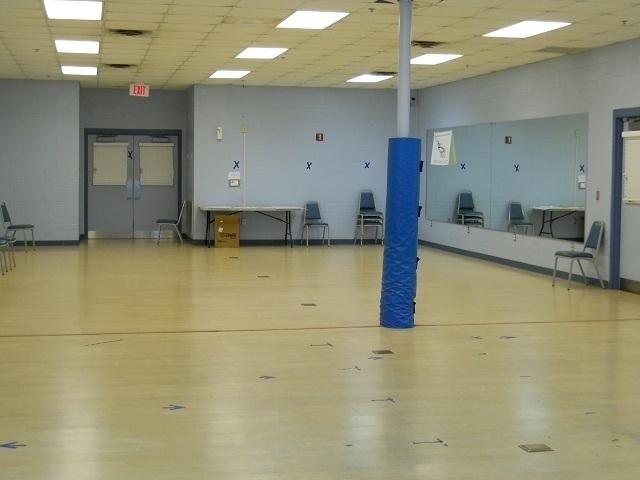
[424,112,589,244]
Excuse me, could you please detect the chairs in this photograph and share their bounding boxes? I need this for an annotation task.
[552,219,604,290]
[0,201,35,253]
[507,201,534,236]
[456,190,484,228]
[155,200,185,246]
[353,188,384,246]
[0,236,18,275]
[300,202,331,247]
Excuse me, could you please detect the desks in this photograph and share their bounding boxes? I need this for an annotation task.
[200,204,302,248]
[530,206,585,238]
